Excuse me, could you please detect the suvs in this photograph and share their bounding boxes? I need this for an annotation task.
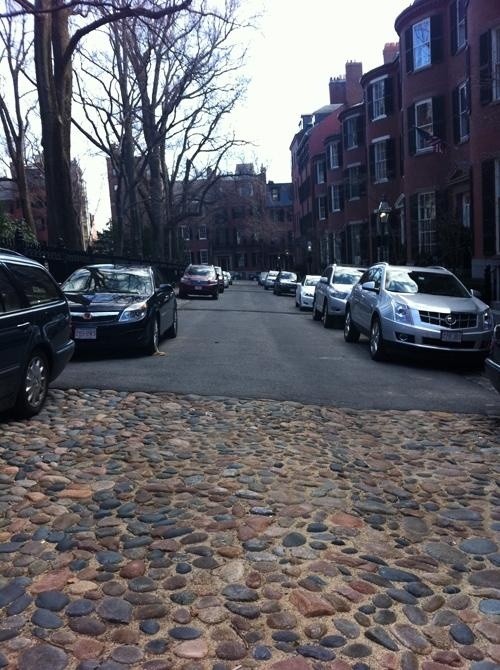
[0,246,76,421]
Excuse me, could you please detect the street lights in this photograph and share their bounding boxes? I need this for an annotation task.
[278,256,281,271]
[306,241,313,275]
[377,199,393,262]
[285,250,289,270]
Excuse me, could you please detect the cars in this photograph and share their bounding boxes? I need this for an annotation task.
[60,261,180,355]
[179,263,233,300]
[249,261,496,371]
